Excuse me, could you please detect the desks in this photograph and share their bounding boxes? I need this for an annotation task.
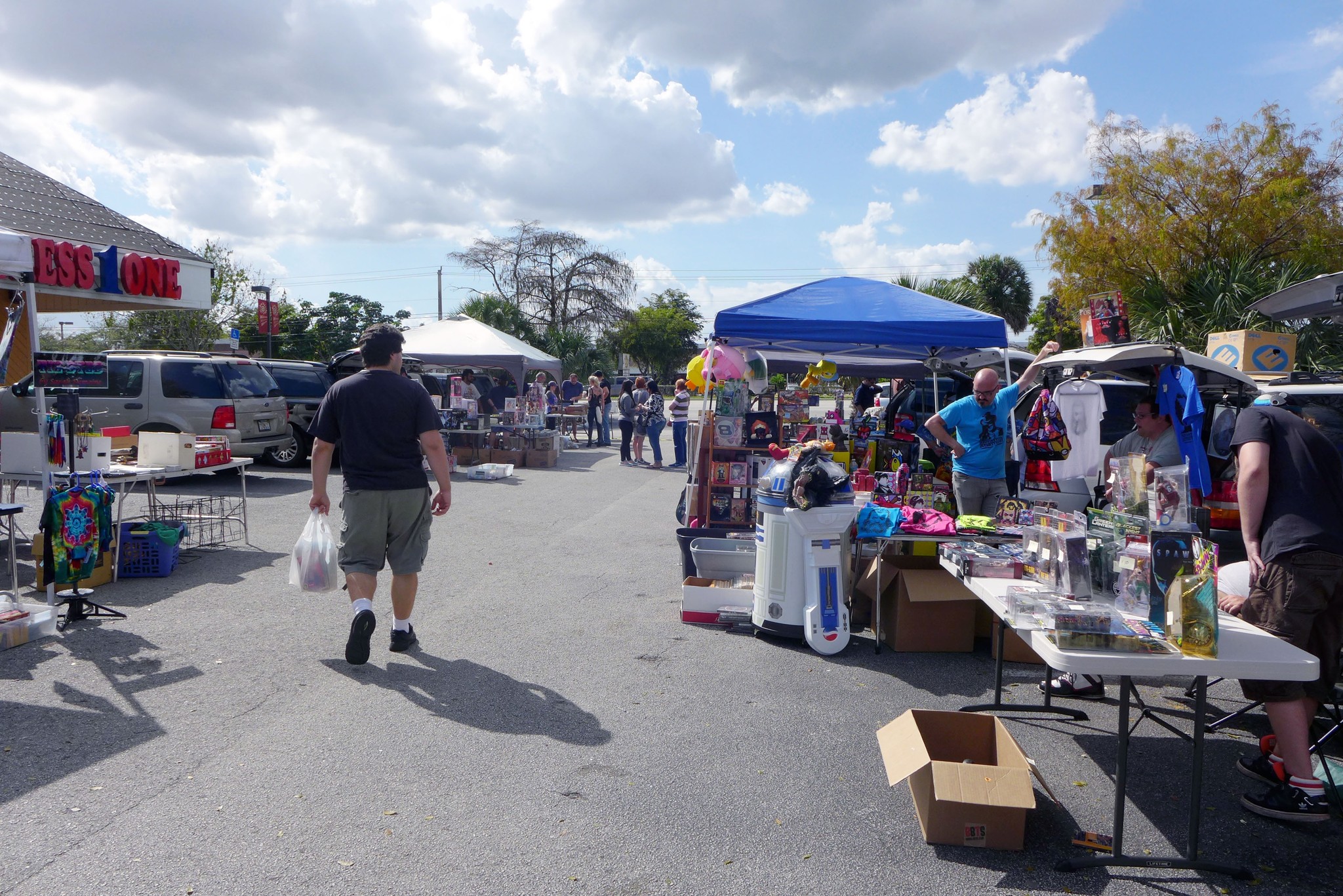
[439,426,491,468]
[940,547,1322,874]
[1,453,254,582]
[489,423,546,450]
[545,414,591,443]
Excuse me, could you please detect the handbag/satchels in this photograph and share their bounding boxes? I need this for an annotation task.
[635,424,647,436]
[632,419,637,428]
[288,507,338,593]
[589,394,596,406]
[638,412,649,427]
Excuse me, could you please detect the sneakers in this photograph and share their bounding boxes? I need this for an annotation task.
[668,462,683,467]
[389,622,416,651]
[345,609,376,664]
[601,441,611,446]
[637,458,650,465]
[594,441,598,445]
[1038,670,1105,699]
[619,459,632,466]
[627,459,639,465]
[1236,754,1286,785]
[1236,788,1333,822]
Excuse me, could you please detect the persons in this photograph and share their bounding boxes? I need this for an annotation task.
[304,324,452,666]
[1036,560,1253,700]
[481,372,689,513]
[399,367,409,380]
[1227,390,1343,825]
[1102,395,1183,506]
[924,340,1061,518]
[1002,415,1029,498]
[460,368,484,448]
[1154,475,1180,521]
[1083,314,1094,347]
[1093,299,1116,319]
[1123,558,1151,602]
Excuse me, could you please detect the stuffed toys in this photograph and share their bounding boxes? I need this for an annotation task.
[685,338,769,397]
[799,358,839,389]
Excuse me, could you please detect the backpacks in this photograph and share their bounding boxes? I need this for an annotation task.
[1021,375,1073,460]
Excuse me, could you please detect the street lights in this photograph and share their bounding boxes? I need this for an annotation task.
[251,285,272,359]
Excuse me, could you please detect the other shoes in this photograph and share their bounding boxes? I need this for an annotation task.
[573,438,579,442]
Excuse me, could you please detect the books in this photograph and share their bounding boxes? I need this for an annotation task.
[707,574,755,592]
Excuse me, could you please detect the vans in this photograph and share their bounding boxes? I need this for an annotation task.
[584,375,654,399]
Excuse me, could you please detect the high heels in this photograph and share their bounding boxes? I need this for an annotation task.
[597,441,601,447]
[586,442,592,447]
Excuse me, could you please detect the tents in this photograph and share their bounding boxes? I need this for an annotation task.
[345,312,563,436]
[683,275,1015,457]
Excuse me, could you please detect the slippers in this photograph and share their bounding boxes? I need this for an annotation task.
[647,463,660,469]
[650,462,662,468]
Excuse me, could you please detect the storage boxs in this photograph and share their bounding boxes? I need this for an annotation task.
[194,448,231,468]
[0,431,111,474]
[100,425,131,437]
[136,429,196,470]
[451,446,491,466]
[446,454,457,473]
[526,448,557,468]
[990,611,1049,664]
[855,552,985,654]
[875,709,1061,852]
[667,380,942,621]
[1207,328,1296,377]
[535,435,561,457]
[490,448,524,467]
[112,520,188,578]
[507,435,534,451]
[110,433,138,449]
[466,463,514,480]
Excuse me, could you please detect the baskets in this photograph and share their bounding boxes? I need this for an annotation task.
[110,521,185,576]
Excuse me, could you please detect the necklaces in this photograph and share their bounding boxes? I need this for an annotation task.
[74,413,94,459]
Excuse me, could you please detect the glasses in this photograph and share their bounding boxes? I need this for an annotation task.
[972,385,998,396]
[868,379,876,384]
[1132,412,1157,419]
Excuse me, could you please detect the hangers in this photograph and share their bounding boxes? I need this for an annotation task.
[67,472,83,492]
[1058,368,1102,396]
[90,471,97,488]
[92,470,102,486]
[99,468,108,486]
[1167,344,1182,372]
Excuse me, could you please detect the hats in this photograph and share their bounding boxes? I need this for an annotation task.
[895,419,916,430]
[548,380,558,387]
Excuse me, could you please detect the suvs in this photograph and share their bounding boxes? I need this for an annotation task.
[250,347,427,466]
[421,372,505,416]
[870,270,1343,563]
[0,349,294,487]
